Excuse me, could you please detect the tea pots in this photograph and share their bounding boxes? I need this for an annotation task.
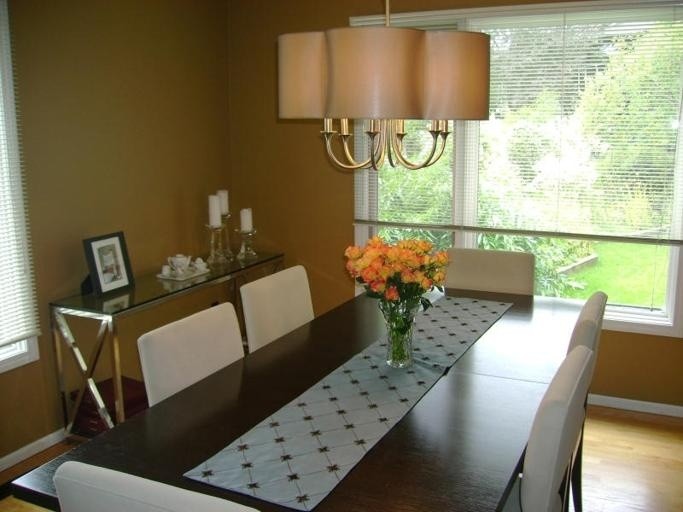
[167,253,191,275]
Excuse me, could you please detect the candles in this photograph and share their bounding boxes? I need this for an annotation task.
[216,190,229,216]
[208,195,222,227]
[240,208,253,233]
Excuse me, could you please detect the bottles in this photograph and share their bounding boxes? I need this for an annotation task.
[203,211,258,265]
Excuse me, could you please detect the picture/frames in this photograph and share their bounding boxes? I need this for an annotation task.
[84,230,135,297]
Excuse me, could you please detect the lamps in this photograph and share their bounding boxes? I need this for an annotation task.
[278,0,490,171]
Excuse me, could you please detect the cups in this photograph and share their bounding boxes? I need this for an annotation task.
[194,257,203,273]
[161,265,170,278]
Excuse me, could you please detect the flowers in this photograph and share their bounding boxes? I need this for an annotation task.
[345,235,451,365]
